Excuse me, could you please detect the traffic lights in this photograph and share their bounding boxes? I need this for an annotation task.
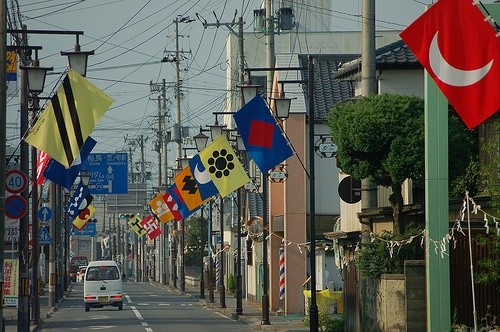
[120,213,135,219]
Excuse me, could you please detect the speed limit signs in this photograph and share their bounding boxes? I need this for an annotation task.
[4,170,28,194]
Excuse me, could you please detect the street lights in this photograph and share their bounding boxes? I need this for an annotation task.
[172,113,270,325]
[1,29,93,332]
[238,55,319,332]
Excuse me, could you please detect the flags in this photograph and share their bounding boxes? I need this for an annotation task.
[23,67,114,232]
[233,95,294,177]
[399,0,500,129]
[127,133,251,240]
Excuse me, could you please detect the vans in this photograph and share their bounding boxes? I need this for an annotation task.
[84,261,123,311]
